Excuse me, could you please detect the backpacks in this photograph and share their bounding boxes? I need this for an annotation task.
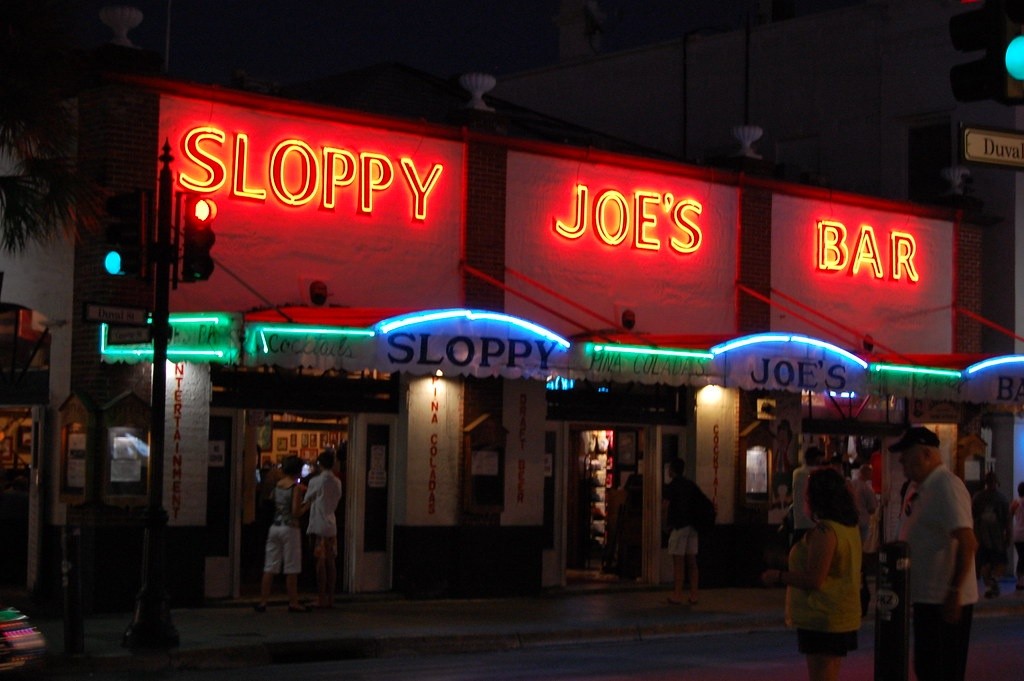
[680,481,716,530]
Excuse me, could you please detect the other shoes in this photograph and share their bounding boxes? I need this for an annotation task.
[984,592,993,598]
[690,598,698,608]
[990,576,1000,595]
[667,597,679,604]
[289,606,313,612]
[255,606,266,612]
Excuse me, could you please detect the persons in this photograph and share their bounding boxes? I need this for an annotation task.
[265,439,348,539]
[879,427,977,681]
[820,439,885,618]
[792,444,825,548]
[302,448,342,607]
[253,456,319,613]
[663,456,701,608]
[1010,480,1024,590]
[973,471,1012,598]
[761,466,864,681]
[256,461,275,568]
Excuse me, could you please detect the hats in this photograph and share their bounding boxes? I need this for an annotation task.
[888,427,939,452]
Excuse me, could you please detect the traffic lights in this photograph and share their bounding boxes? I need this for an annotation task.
[184,196,219,282]
[102,190,142,278]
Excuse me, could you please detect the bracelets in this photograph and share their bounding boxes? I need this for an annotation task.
[778,569,785,583]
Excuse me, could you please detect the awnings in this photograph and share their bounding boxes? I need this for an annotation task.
[91,292,1024,427]
